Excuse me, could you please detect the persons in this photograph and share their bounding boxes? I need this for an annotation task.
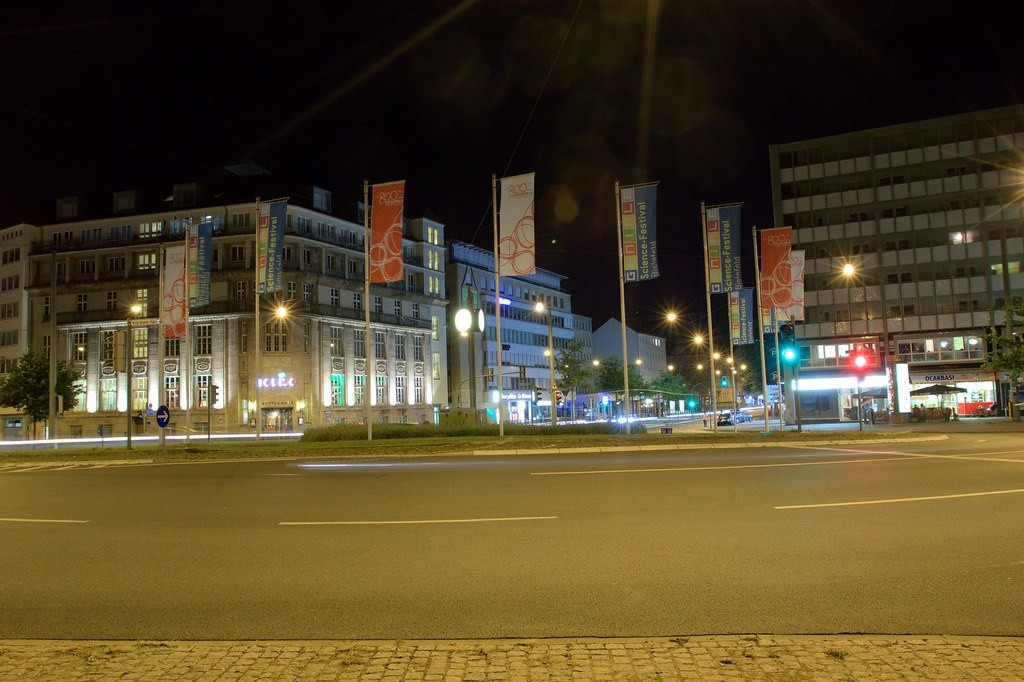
[983,402,997,416]
[702,411,708,428]
[913,403,925,413]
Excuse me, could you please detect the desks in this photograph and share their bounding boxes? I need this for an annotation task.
[977,409,986,417]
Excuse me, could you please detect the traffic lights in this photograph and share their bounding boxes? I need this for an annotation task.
[779,323,798,366]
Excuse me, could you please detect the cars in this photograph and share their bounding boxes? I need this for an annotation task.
[716,411,753,427]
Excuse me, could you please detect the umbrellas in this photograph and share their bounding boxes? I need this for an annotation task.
[909,384,967,409]
[852,387,887,408]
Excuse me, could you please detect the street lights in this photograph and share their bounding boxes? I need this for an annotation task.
[843,263,857,338]
[454,287,487,438]
[536,295,558,428]
[854,356,865,432]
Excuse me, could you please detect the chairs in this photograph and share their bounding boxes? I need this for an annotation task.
[969,409,977,418]
